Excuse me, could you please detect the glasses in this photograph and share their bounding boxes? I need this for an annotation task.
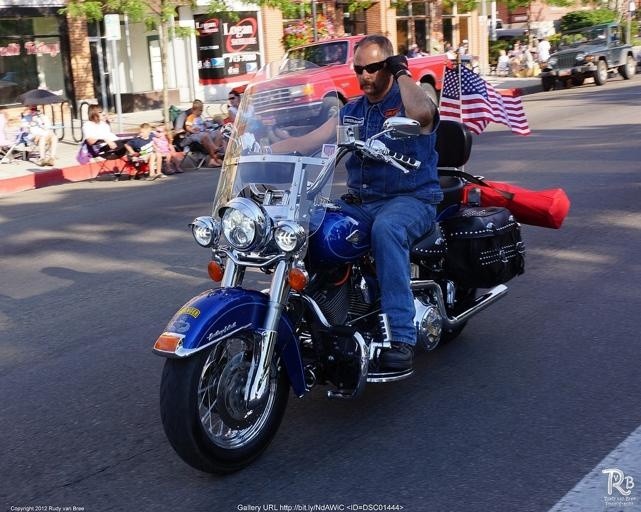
[354,60,386,74]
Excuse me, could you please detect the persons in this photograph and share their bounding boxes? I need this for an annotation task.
[227,90,279,145]
[245,33,445,372]
[82,103,149,181]
[174,99,223,167]
[152,126,184,175]
[185,101,221,152]
[123,122,167,180]
[0,110,19,164]
[20,104,59,166]
[406,35,558,78]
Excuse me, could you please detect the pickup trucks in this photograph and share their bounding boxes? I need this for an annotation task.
[249,37,456,133]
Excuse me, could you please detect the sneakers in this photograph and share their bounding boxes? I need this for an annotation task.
[379,341,414,371]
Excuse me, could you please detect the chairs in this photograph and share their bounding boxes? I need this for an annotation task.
[0,124,210,181]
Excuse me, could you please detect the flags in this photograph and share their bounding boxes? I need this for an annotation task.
[460,64,531,136]
[439,66,490,135]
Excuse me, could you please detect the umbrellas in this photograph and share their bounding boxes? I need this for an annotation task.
[13,87,71,112]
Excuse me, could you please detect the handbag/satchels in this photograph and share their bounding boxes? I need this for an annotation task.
[439,206,526,288]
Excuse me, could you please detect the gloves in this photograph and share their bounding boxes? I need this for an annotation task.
[386,54,412,79]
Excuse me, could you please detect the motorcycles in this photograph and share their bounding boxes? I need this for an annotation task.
[153,58,524,475]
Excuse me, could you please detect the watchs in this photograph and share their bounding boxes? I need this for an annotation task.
[395,69,413,82]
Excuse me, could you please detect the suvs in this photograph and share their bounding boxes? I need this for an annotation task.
[540,22,636,92]
